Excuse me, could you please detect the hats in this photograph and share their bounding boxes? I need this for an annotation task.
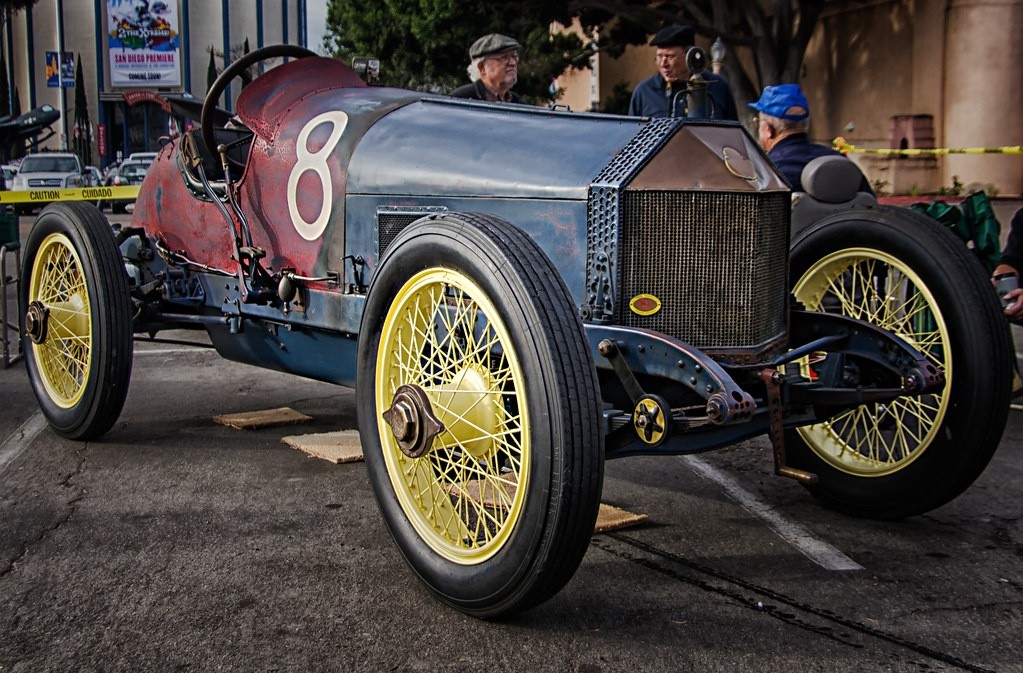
[650,24,695,46]
[747,84,810,120]
[469,33,522,60]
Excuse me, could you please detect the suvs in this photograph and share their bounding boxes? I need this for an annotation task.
[10,151,92,216]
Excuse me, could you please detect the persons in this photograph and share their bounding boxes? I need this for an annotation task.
[749,86,876,202]
[991,207,1023,326]
[629,25,739,121]
[451,32,528,105]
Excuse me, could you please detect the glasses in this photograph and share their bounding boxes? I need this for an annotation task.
[653,55,674,61]
[752,117,768,127]
[486,52,519,63]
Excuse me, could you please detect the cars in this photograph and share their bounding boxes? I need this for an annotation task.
[0,158,24,216]
[86,152,161,215]
